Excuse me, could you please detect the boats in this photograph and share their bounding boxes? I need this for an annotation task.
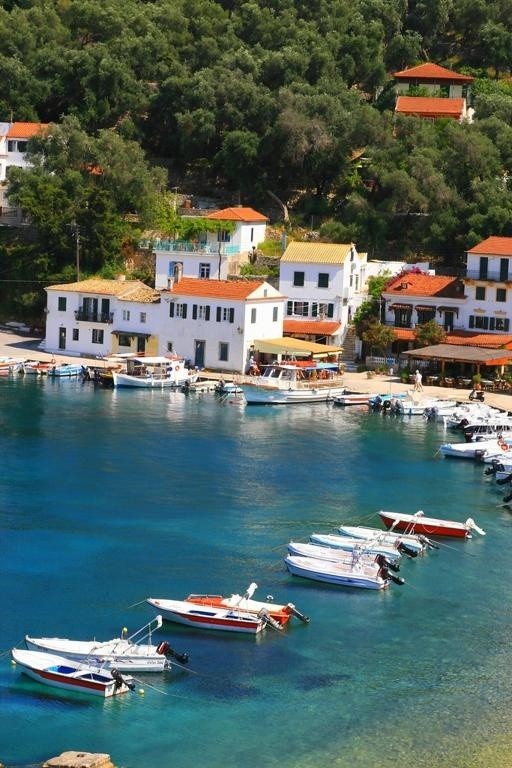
[284,508,486,590]
[8,645,137,697]
[183,582,313,627]
[144,596,286,635]
[330,392,512,481]
[0,346,244,395]
[24,614,188,671]
[233,353,347,404]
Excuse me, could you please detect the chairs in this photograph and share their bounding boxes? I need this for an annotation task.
[438,376,465,389]
[482,379,508,392]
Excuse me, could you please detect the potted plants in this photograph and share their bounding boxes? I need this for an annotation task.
[379,366,384,375]
[400,369,408,383]
[473,373,481,391]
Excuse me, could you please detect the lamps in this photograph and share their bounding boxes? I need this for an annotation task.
[237,327,243,334]
[43,307,49,313]
[74,311,79,319]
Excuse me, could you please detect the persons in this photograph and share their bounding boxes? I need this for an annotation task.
[413,369,424,392]
[248,356,260,372]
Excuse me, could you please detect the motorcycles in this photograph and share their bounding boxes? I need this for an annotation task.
[469,387,485,401]
